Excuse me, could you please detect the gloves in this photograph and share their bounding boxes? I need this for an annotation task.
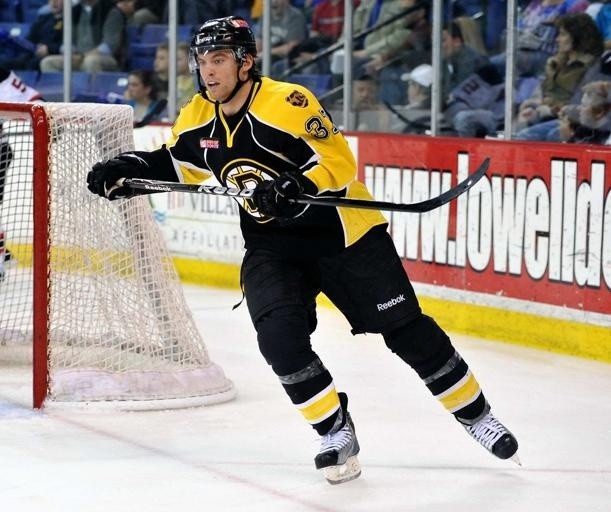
[254,171,318,220]
[87,158,141,200]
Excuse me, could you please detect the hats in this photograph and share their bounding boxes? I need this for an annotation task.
[399,64,432,87]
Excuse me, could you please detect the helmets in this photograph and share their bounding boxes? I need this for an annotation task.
[191,16,257,63]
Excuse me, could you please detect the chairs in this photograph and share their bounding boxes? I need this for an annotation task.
[2,2,611,144]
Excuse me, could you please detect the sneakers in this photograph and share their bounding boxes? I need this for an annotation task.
[464,413,518,459]
[315,415,359,468]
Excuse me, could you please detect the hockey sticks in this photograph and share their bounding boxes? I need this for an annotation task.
[7,99,168,138]
[380,95,558,139]
[113,157,491,213]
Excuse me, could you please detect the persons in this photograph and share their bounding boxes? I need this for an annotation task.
[440,2,610,145]
[250,2,433,131]
[0,52,45,282]
[0,0,156,76]
[85,13,519,473]
[108,45,199,128]
